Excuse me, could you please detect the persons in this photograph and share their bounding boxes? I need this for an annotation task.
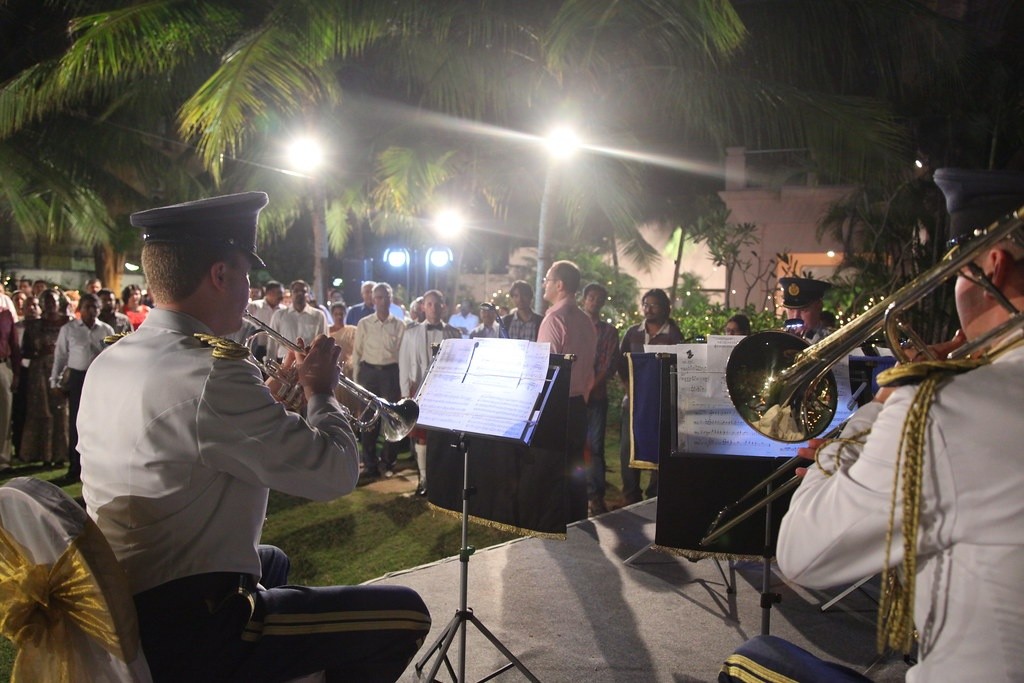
[582,280,619,516]
[75,191,433,683]
[613,288,684,510]
[779,278,837,332]
[0,277,543,483]
[725,314,750,336]
[716,164,1024,683]
[536,259,598,523]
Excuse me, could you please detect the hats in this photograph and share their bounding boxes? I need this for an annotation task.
[778,278,833,309]
[932,167,1024,249]
[130,191,269,270]
[479,302,493,311]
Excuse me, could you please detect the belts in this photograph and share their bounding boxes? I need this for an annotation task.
[0,356,11,363]
[363,361,397,371]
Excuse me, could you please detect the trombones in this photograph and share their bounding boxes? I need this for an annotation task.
[726,202,1024,444]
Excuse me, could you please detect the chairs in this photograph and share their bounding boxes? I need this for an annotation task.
[0,476,154,683]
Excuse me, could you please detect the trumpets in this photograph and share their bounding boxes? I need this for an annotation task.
[245,311,422,442]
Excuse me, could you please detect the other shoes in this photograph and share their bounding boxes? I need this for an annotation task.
[52,462,64,470]
[613,500,629,509]
[359,465,380,480]
[380,459,397,468]
[38,461,51,470]
[591,498,609,517]
[63,472,79,482]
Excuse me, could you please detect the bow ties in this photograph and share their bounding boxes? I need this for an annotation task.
[427,322,442,330]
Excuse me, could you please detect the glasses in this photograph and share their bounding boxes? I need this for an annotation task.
[642,303,660,309]
